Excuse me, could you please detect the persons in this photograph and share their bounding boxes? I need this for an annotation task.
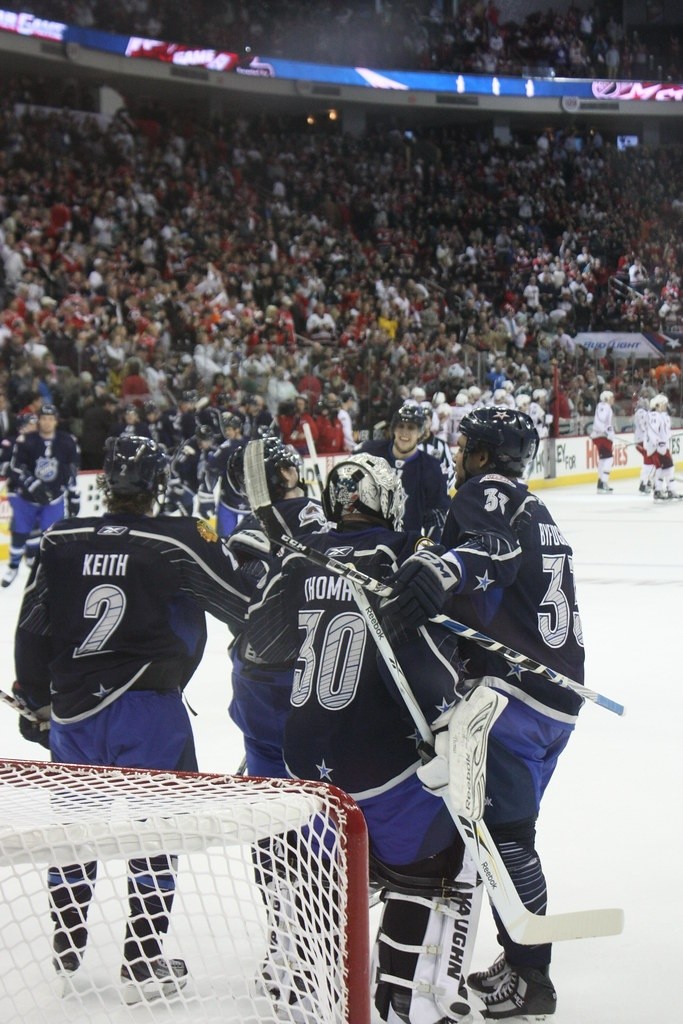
[352,403,450,540]
[441,0,683,82]
[334,123,683,449]
[240,451,485,1023]
[416,408,457,491]
[11,435,259,1003]
[213,433,336,994]
[632,387,683,505]
[0,71,344,476]
[0,0,455,75]
[374,402,594,1022]
[591,390,616,493]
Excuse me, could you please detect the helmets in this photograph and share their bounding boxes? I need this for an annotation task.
[458,409,540,476]
[40,404,59,421]
[387,406,433,440]
[198,407,220,426]
[143,401,157,415]
[14,413,37,429]
[247,394,263,406]
[183,390,197,401]
[123,404,138,420]
[322,453,407,524]
[227,435,303,505]
[225,417,244,434]
[104,436,168,498]
[217,393,231,405]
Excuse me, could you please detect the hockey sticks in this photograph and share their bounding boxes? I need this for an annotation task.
[244,437,625,716]
[0,689,41,722]
[338,562,624,945]
[303,422,325,489]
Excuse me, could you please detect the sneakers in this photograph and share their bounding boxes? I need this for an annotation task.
[480,971,558,1024]
[122,958,191,1005]
[597,478,682,500]
[53,951,83,998]
[467,952,510,994]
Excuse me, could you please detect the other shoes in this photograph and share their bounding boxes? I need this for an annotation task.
[257,952,317,1003]
[1,564,19,587]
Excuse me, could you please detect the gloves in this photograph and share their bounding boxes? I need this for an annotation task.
[69,490,81,517]
[23,476,50,504]
[388,544,459,629]
[19,712,47,750]
